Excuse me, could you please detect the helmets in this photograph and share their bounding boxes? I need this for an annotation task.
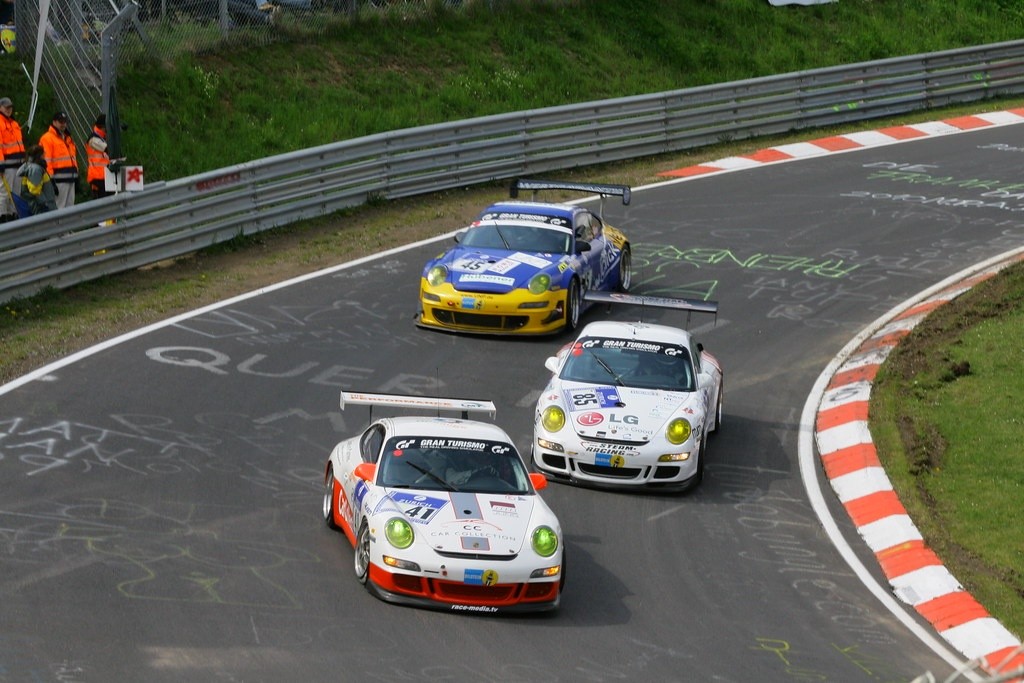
[654,353,679,365]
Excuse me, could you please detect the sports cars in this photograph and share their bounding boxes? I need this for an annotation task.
[410,178,633,340]
[320,392,567,617]
[531,288,724,497]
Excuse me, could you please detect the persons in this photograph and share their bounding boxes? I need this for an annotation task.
[86,113,112,227]
[639,353,684,383]
[445,449,499,491]
[0,97,26,225]
[19,145,60,244]
[38,112,80,237]
[226,0,465,28]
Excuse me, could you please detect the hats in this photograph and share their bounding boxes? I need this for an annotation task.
[0,97,13,107]
[54,112,69,123]
[96,115,106,124]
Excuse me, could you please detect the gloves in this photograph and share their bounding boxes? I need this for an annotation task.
[0,164,5,174]
[75,178,80,185]
[54,184,59,196]
